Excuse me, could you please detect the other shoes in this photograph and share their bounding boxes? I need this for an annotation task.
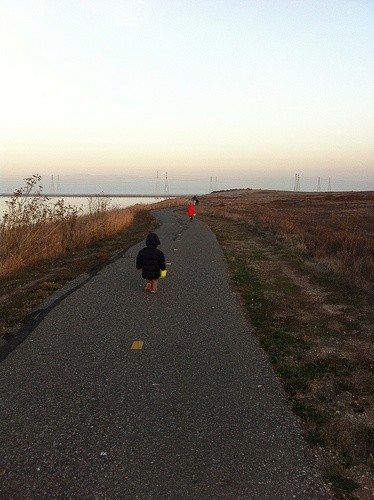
[145,282,151,292]
[151,290,157,293]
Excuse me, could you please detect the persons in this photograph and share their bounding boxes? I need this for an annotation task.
[192,195,200,215]
[136,231,166,293]
[187,203,196,220]
